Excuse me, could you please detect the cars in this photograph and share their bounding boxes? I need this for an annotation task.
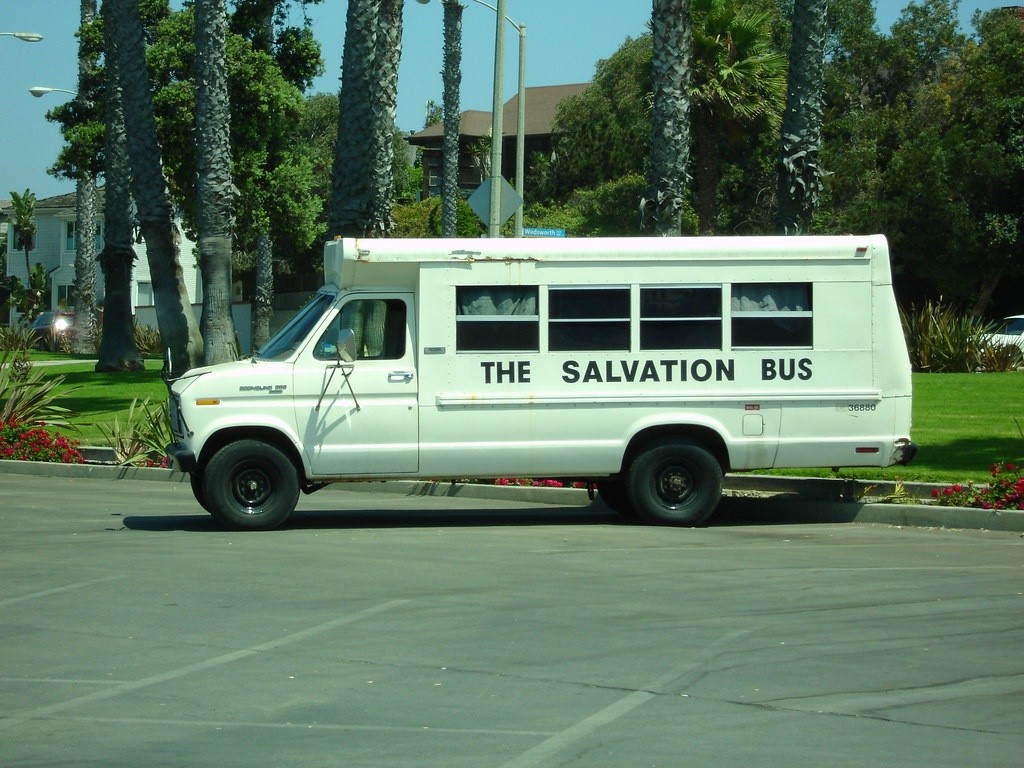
[966,314,1024,365]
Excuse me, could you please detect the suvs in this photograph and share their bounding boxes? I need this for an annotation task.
[28,310,98,353]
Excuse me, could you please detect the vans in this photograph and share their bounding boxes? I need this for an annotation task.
[157,231,919,533]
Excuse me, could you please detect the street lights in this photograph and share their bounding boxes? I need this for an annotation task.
[415,0,527,237]
